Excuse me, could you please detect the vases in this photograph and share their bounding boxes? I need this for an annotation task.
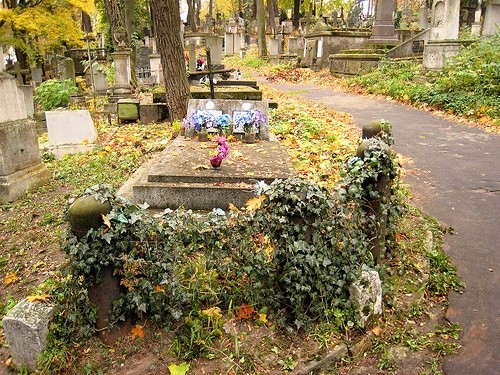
[196,63,202,71]
[197,129,209,142]
[243,130,257,144]
[210,156,222,170]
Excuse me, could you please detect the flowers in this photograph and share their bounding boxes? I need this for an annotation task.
[208,136,229,164]
[183,108,269,134]
[197,58,205,64]
